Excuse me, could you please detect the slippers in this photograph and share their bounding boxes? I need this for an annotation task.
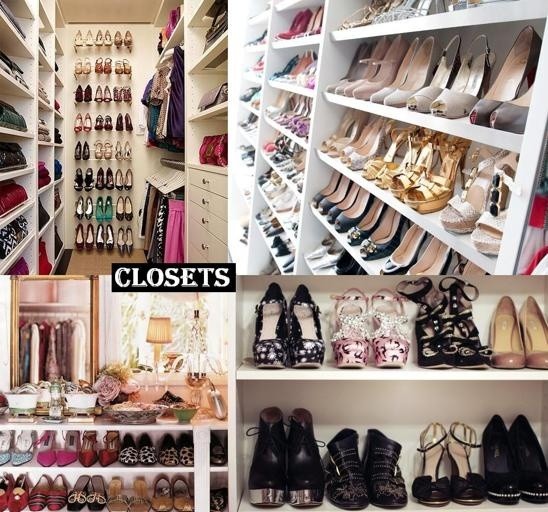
[68,471,150,512]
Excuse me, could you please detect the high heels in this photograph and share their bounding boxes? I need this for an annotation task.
[411,421,451,508]
[73,25,137,256]
[325,427,370,511]
[287,408,324,509]
[519,295,548,371]
[330,285,370,369]
[509,413,547,504]
[253,282,289,370]
[395,276,459,373]
[437,276,491,372]
[242,403,287,510]
[480,413,523,506]
[238,6,540,272]
[371,287,411,369]
[487,288,525,370]
[289,283,326,370]
[446,410,488,509]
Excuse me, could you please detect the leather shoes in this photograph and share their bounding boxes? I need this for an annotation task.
[0,430,231,467]
[150,473,196,510]
[1,474,66,511]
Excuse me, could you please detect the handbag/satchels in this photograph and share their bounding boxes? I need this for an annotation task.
[1,179,30,220]
[0,256,34,276]
[54,186,63,213]
[0,97,30,138]
[195,132,230,169]
[197,78,230,113]
[39,236,53,275]
[54,224,64,261]
[0,140,32,172]
[38,193,52,231]
[0,216,32,261]
[201,0,233,55]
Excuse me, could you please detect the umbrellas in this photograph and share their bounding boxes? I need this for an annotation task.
[0,430,231,467]
[1,474,66,511]
[150,473,196,510]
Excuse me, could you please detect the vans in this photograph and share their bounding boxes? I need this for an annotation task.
[395,276,459,373]
[253,282,289,370]
[487,288,525,370]
[480,413,523,506]
[437,276,491,372]
[446,410,488,509]
[519,295,548,371]
[287,408,324,509]
[509,413,547,504]
[289,283,326,370]
[330,285,370,369]
[371,287,411,369]
[411,421,451,508]
[325,427,370,511]
[73,25,137,256]
[242,403,287,510]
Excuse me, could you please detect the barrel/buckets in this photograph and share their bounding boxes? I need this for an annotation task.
[68,471,150,512]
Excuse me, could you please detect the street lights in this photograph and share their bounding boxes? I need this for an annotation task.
[195,132,230,169]
[1,179,30,220]
[0,256,34,276]
[54,224,64,261]
[197,78,230,113]
[38,193,52,231]
[0,140,32,172]
[54,186,63,213]
[0,216,32,261]
[39,236,53,275]
[201,0,233,55]
[0,97,30,138]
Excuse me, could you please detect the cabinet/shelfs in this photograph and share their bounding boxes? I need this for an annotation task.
[0,0,548,511]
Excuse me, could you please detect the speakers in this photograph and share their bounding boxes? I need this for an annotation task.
[361,427,409,510]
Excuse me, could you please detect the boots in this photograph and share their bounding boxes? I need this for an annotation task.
[361,427,409,510]
